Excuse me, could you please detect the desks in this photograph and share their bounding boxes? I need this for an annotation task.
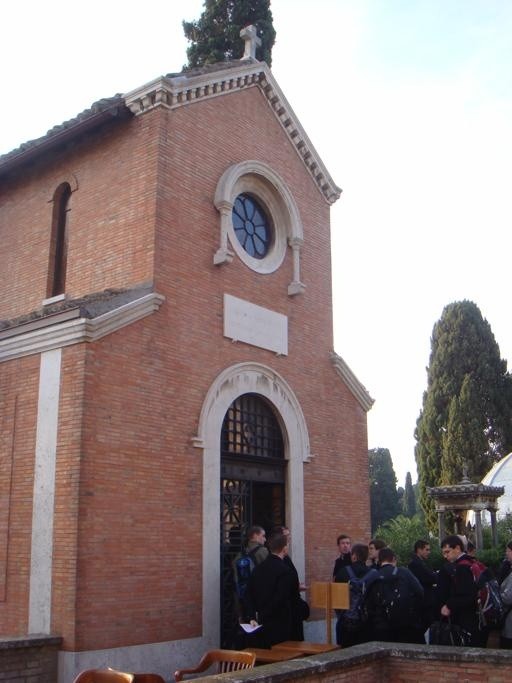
[273,639,341,657]
[240,648,304,667]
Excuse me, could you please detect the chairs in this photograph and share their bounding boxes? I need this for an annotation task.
[108,666,166,683]
[73,667,135,683]
[174,649,257,683]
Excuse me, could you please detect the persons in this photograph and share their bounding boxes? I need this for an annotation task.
[332,533,512,647]
[232,525,310,666]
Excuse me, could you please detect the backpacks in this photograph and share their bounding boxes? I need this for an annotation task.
[448,559,504,629]
[358,567,411,631]
[340,566,377,628]
[235,544,262,605]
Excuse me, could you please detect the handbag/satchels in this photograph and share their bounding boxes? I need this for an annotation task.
[424,615,471,647]
